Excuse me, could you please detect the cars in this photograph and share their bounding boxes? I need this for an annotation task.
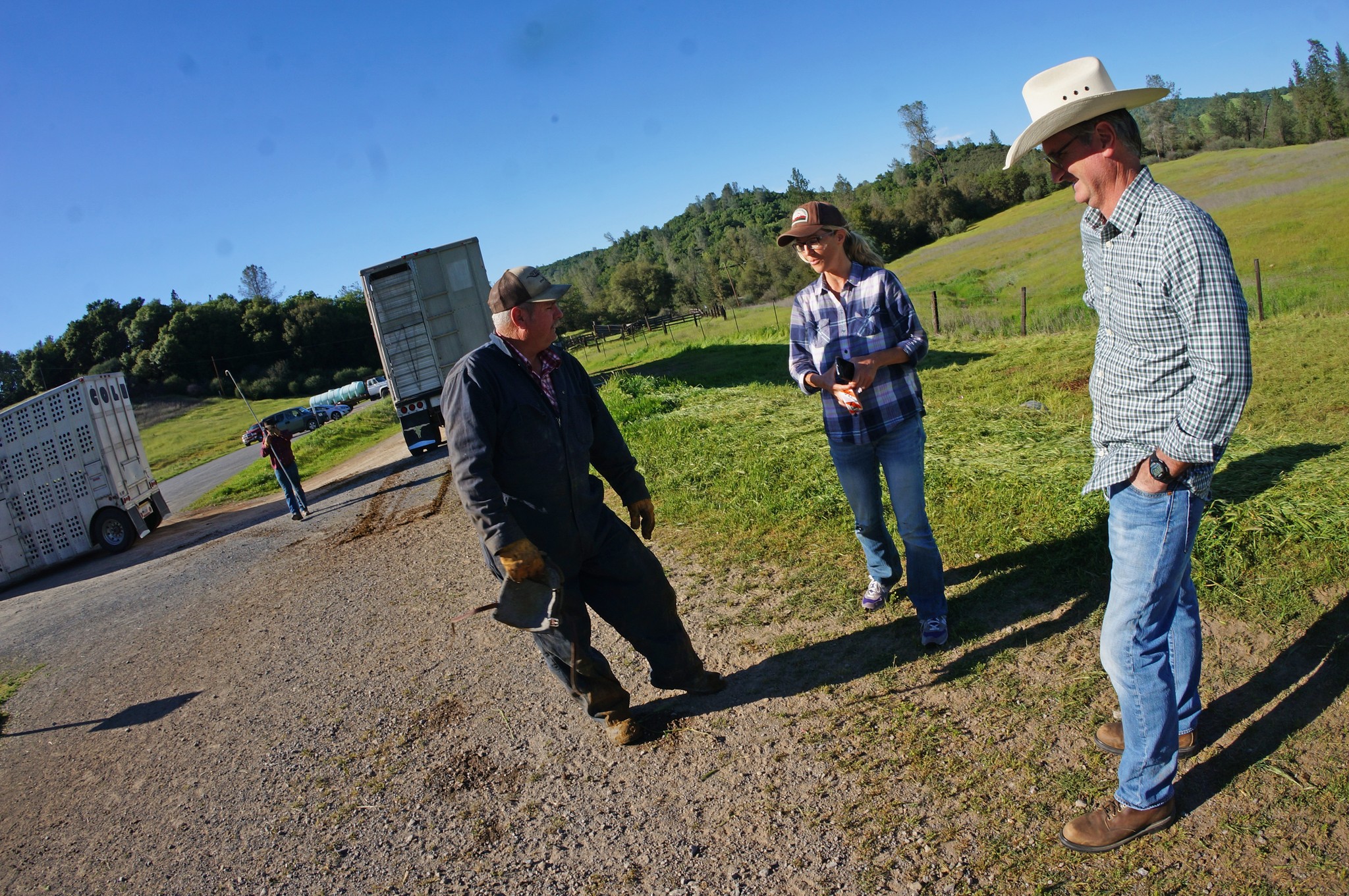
[294,404,351,426]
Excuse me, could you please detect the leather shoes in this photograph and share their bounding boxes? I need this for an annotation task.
[1094,721,1201,757]
[1059,796,1178,852]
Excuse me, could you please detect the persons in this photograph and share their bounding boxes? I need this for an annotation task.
[439,264,725,743]
[1001,58,1252,854]
[781,201,953,649]
[258,418,311,520]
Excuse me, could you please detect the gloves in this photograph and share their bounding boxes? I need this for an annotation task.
[265,425,281,437]
[626,499,656,540]
[264,436,272,448]
[497,537,546,583]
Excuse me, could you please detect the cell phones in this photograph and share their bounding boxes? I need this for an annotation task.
[835,356,855,382]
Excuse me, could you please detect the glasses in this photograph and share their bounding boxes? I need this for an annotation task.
[264,425,275,430]
[1044,126,1098,166]
[791,231,836,252]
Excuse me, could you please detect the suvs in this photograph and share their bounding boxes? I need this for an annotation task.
[242,422,263,447]
[261,406,330,434]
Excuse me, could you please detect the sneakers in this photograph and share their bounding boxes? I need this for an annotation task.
[862,569,901,609]
[918,615,948,646]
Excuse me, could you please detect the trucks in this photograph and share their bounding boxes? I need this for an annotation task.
[0,371,171,593]
[358,236,495,457]
[332,376,389,411]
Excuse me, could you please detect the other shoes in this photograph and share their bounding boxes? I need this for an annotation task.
[605,713,638,745]
[651,668,724,694]
[291,512,303,520]
[302,509,310,516]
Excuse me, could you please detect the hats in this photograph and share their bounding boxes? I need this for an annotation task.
[1002,56,1168,170]
[776,201,847,247]
[263,418,279,426]
[488,266,572,314]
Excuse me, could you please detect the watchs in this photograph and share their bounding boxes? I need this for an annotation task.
[1149,451,1176,490]
